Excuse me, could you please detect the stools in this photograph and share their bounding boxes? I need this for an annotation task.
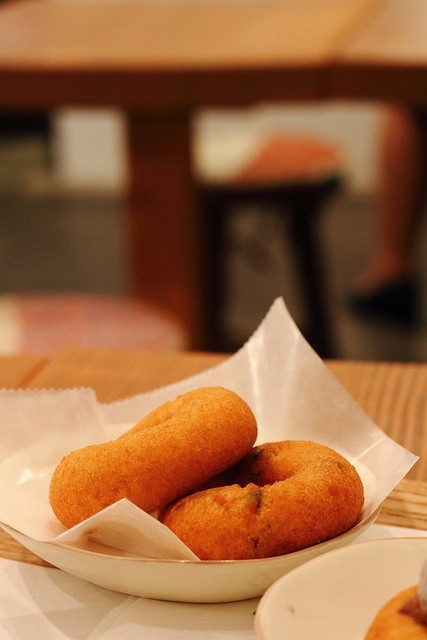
[186,134,346,362]
[2,291,186,353]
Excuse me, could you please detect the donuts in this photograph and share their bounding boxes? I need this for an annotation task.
[365,583,427,639]
[49,386,258,530]
[156,440,365,560]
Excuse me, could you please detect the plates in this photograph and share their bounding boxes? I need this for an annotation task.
[0,423,384,602]
[252,537,427,639]
